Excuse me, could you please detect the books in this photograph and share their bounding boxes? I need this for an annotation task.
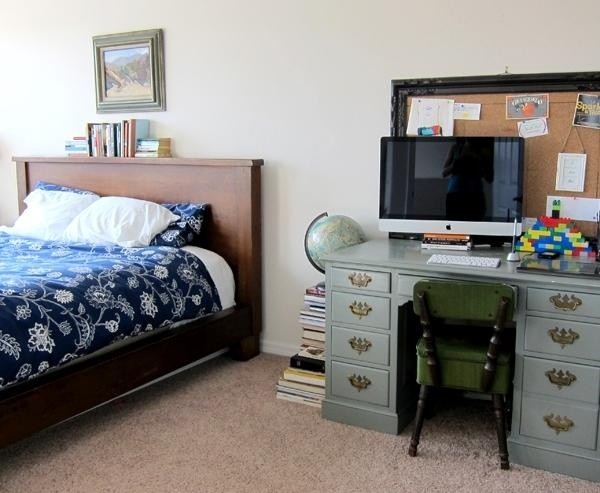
[63,117,172,158]
[275,284,326,408]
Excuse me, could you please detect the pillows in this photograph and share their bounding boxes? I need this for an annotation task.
[11,179,213,247]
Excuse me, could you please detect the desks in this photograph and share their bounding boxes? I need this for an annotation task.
[317,236,600,482]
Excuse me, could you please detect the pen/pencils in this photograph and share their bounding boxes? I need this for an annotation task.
[512,217,518,253]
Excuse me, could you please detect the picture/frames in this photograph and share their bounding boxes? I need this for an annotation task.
[90,28,167,114]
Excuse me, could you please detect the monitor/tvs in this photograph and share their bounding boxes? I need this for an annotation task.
[376,135,524,251]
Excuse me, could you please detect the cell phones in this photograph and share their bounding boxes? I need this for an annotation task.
[538,249,560,259]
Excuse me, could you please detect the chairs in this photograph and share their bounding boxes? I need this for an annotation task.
[408,278,515,471]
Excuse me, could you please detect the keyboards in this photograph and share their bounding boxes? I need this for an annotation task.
[425,253,501,270]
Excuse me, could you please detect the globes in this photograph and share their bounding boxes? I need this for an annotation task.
[304,212,368,292]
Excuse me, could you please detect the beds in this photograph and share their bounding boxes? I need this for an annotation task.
[0,155,264,450]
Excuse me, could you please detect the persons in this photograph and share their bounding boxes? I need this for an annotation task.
[440,140,495,218]
